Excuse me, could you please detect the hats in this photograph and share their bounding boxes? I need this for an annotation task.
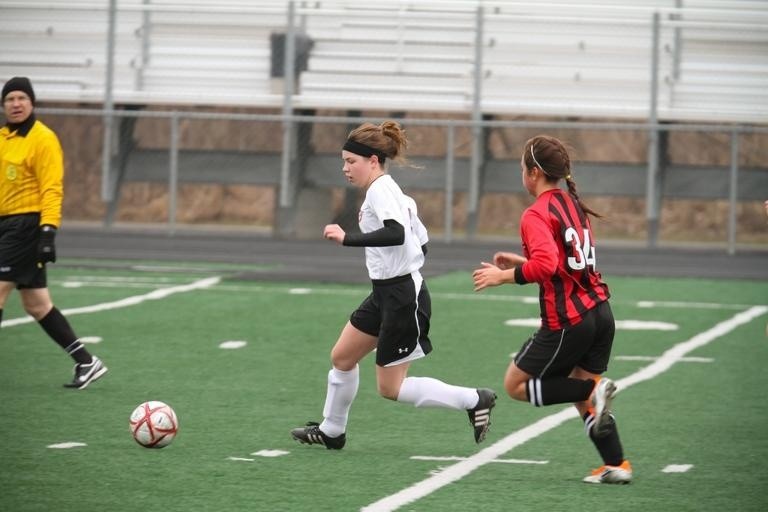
[1,77,35,107]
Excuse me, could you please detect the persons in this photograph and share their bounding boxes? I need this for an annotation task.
[1,74,110,391]
[471,133,635,487]
[286,118,500,452]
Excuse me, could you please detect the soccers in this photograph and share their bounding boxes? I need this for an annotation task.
[130,401,179,448]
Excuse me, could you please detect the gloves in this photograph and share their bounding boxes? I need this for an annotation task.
[34,225,56,268]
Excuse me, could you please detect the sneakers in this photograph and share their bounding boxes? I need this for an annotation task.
[64,355,107,389]
[582,459,632,485]
[291,421,346,450]
[587,377,617,430]
[466,388,497,443]
